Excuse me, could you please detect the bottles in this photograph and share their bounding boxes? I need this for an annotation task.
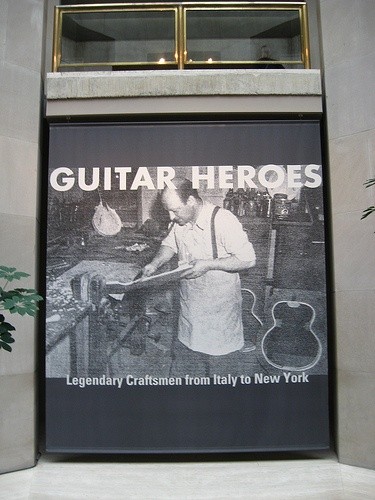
[223,188,268,218]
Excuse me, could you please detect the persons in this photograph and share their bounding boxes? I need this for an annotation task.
[138,176,256,380]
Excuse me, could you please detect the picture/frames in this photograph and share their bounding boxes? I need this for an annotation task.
[37,112,340,464]
